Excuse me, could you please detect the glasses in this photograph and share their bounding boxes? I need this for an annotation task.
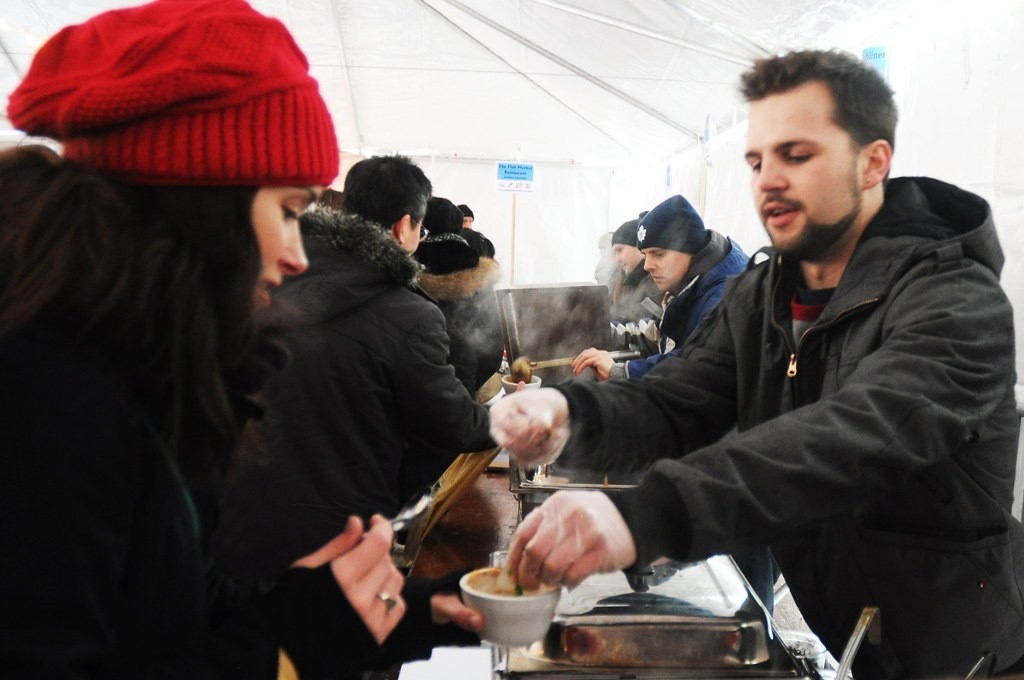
[412,215,430,243]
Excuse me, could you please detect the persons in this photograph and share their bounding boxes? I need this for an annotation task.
[486,51,1022,680]
[605,209,669,362]
[226,155,519,565]
[569,194,781,621]
[1,0,487,680]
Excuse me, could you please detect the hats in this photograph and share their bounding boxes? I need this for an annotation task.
[423,197,462,238]
[9,0,341,187]
[456,204,474,220]
[635,194,708,254]
[611,212,652,247]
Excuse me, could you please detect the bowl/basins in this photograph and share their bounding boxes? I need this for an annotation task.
[458,567,561,647]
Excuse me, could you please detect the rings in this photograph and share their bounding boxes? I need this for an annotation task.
[376,592,396,611]
